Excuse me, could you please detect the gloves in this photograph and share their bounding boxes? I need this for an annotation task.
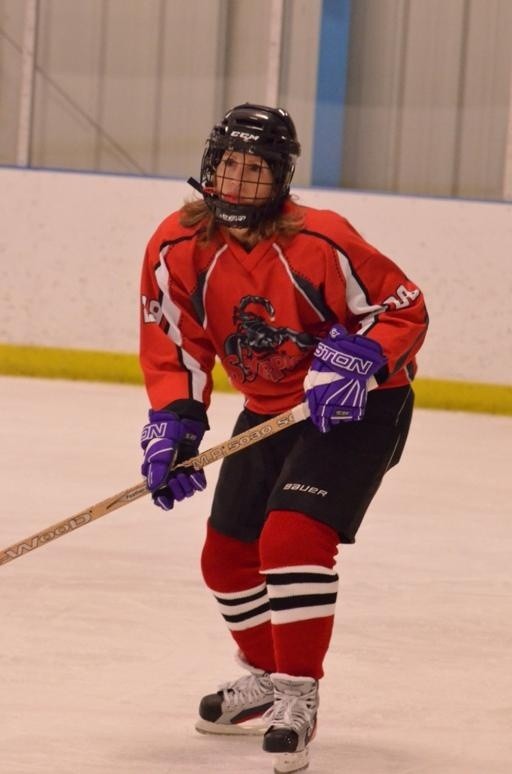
[303,323,388,433]
[141,409,207,511]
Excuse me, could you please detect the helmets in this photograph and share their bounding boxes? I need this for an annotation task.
[188,105,299,227]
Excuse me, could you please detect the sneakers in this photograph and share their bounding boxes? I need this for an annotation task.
[263,673,319,752]
[200,649,274,725]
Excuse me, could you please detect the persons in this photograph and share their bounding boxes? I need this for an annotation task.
[137,103,430,774]
